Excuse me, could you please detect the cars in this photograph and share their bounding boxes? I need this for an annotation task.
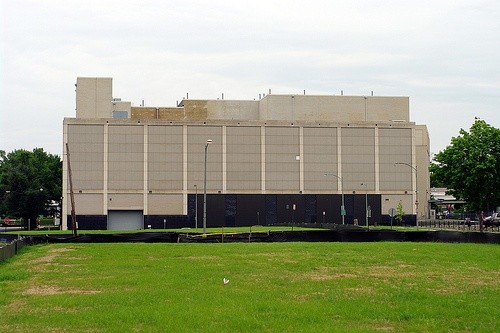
[484,212,500,226]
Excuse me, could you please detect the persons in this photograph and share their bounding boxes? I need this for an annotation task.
[3,216,9,224]
[479,210,486,232]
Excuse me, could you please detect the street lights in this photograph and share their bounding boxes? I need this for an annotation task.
[394,162,419,229]
[361,183,369,227]
[203,140,213,233]
[193,184,198,227]
[323,173,345,225]
